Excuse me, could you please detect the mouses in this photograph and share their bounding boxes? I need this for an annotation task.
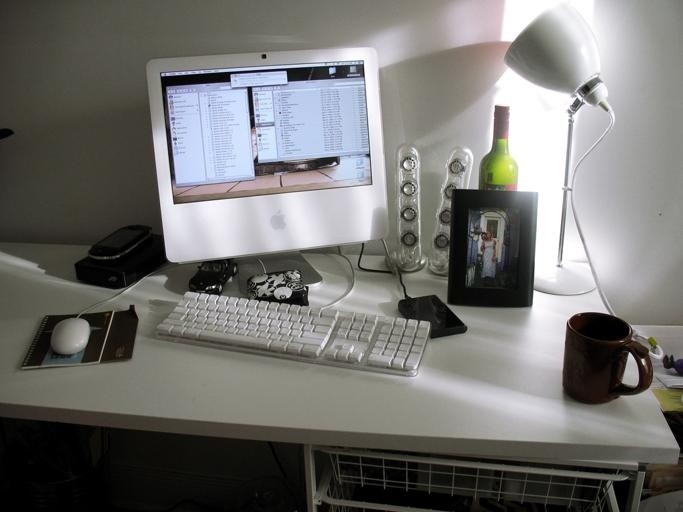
[51,318,91,355]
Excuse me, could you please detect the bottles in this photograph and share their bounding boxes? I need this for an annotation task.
[479,105,519,193]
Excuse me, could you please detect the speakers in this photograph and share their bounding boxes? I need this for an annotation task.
[430,146,474,275]
[395,143,427,271]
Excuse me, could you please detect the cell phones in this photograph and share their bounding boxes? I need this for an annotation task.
[398,295,466,337]
[89,224,151,261]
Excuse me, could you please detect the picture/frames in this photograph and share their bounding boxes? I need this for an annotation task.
[445,187,536,308]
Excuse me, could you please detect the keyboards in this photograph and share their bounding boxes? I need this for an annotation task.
[155,292,431,376]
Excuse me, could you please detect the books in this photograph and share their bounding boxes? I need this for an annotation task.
[638,463,683,495]
[629,324,683,389]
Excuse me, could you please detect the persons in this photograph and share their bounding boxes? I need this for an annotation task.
[480,231,497,279]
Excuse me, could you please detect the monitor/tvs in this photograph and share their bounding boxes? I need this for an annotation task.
[145,48,389,290]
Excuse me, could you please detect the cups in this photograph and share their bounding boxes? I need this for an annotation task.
[562,312,654,404]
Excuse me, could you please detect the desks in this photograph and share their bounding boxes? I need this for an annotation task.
[2,237,680,510]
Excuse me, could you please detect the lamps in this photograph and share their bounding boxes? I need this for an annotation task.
[505,4,617,295]
